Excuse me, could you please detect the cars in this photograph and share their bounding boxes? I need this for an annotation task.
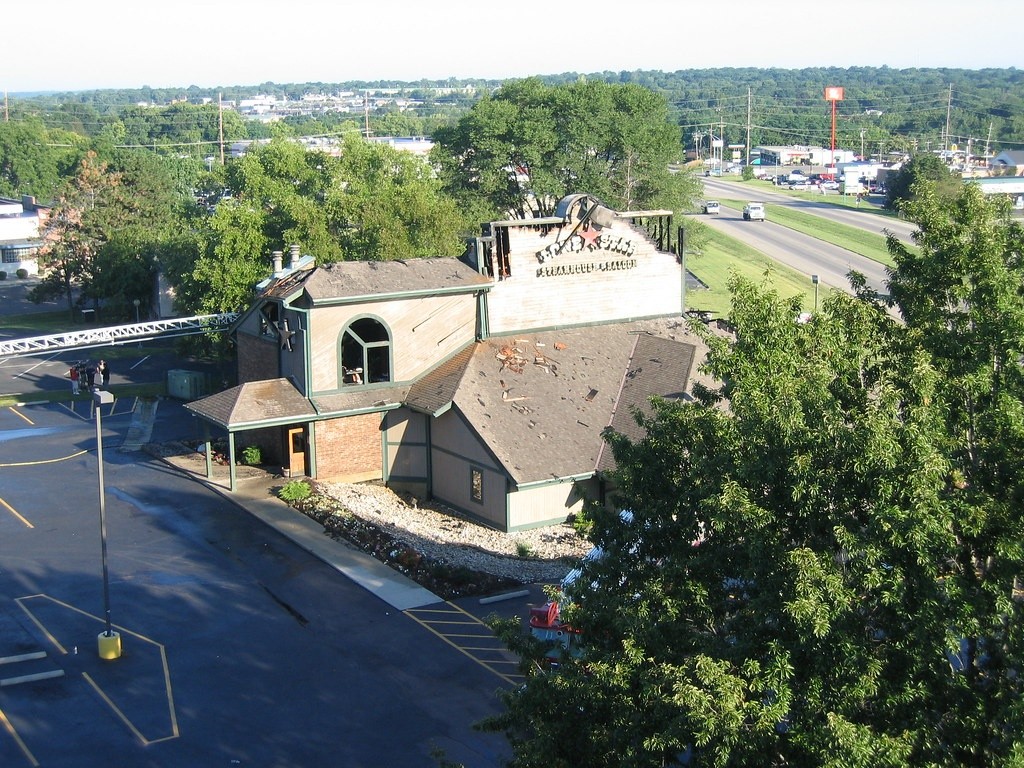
[820,181,839,190]
[871,184,877,191]
[788,180,796,185]
[715,169,720,173]
[705,171,711,176]
[811,179,820,185]
[864,185,872,192]
[792,170,805,175]
[875,187,882,193]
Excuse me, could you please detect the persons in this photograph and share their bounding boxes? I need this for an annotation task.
[70,361,96,395]
[97,360,110,386]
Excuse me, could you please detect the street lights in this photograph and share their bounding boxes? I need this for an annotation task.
[134,299,142,348]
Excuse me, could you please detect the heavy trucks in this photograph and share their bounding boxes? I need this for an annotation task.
[525,495,714,711]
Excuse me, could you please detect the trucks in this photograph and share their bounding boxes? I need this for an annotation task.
[839,182,869,195]
[789,174,806,183]
[743,203,764,222]
[701,201,719,214]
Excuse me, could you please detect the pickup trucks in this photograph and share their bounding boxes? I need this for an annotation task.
[758,174,773,180]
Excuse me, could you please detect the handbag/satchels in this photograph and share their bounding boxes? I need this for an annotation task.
[94,369,103,385]
